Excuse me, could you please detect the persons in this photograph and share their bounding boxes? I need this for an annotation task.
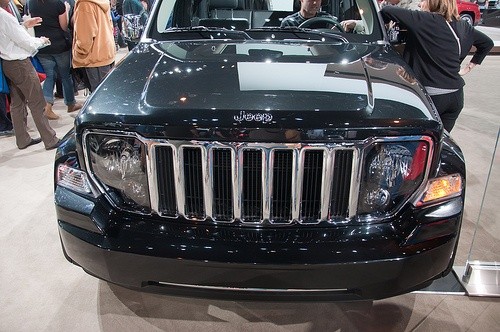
[461,0,500,24]
[340,0,429,61]
[279,0,339,29]
[0,0,156,151]
[377,0,494,134]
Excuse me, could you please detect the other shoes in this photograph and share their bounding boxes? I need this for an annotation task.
[67,104,83,112]
[54,92,64,98]
[43,103,59,120]
[19,138,42,149]
[45,138,63,150]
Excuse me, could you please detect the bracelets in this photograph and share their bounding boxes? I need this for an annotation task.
[466,64,473,70]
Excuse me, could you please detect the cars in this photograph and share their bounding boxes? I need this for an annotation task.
[456,0,480,28]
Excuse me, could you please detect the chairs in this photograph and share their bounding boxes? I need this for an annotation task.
[198,0,295,30]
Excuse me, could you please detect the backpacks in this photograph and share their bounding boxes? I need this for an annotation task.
[120,7,146,42]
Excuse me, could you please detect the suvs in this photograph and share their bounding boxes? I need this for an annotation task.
[54,0,466,302]
